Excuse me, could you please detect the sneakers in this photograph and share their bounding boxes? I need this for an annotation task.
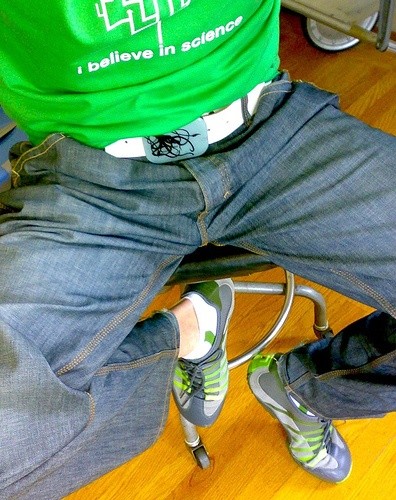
[247,352,353,485]
[171,278,235,428]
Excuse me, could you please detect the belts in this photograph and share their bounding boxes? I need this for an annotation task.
[105,80,272,164]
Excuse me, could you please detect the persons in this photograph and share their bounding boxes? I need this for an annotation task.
[0,0,395,499]
